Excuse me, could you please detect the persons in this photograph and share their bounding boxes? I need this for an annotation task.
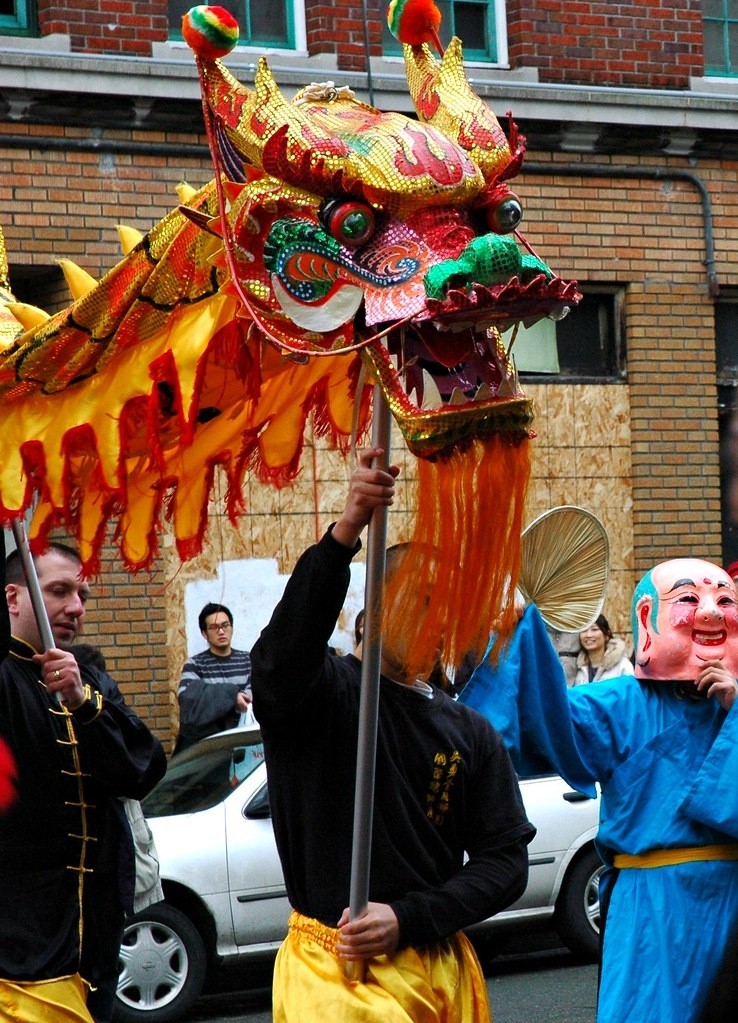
[572,613,637,686]
[171,602,253,812]
[248,446,537,1023]
[0,527,167,1023]
[456,558,738,1023]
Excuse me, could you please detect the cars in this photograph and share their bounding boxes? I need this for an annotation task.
[110,725,607,1023]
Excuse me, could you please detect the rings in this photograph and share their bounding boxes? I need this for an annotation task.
[55,670,60,680]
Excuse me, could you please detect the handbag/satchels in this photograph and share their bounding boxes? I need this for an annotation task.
[229,703,264,783]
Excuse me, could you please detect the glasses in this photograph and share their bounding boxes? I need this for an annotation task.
[206,623,231,632]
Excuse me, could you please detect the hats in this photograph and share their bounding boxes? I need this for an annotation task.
[727,561,738,581]
